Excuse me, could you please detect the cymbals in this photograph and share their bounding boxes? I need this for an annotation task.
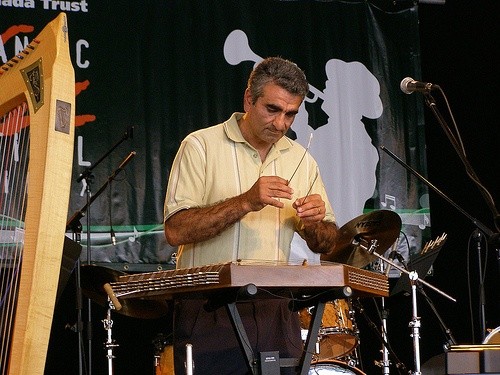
[318,210,403,270]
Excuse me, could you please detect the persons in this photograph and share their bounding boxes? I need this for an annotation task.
[164,57,340,375]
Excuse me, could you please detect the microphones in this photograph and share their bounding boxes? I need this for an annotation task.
[401,77,439,94]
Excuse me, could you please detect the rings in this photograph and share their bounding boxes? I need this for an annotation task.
[318,208,321,213]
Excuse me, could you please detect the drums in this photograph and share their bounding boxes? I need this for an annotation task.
[292,294,358,362]
[305,359,365,375]
